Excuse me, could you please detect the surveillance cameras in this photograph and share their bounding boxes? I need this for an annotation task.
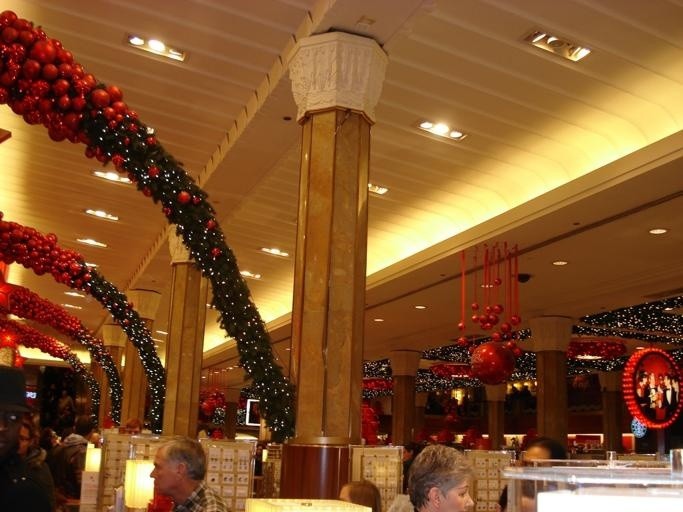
[517,273,530,283]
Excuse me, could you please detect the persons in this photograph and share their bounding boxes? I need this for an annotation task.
[150,438,230,511]
[496,436,568,512]
[0,367,103,511]
[636,369,679,412]
[408,444,474,512]
[340,478,380,512]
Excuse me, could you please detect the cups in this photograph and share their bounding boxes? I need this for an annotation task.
[670,448,683,481]
[606,451,617,467]
